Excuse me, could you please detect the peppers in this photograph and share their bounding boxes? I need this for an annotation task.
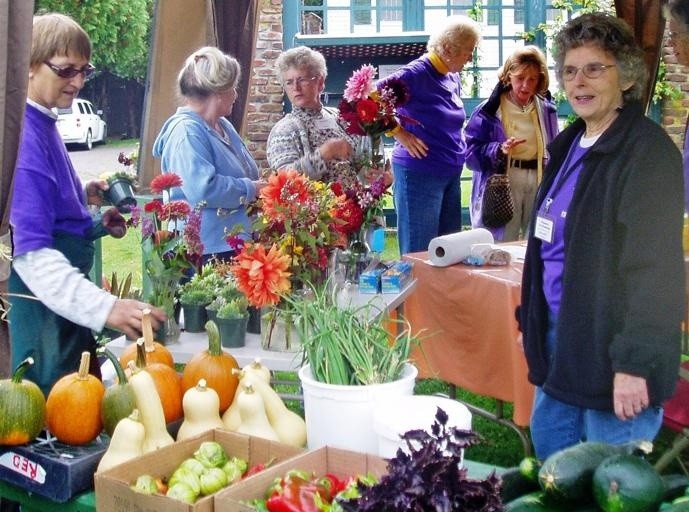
[266,473,347,512]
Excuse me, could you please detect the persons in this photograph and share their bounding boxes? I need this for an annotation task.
[513,11,681,466]
[267,47,373,254]
[5,12,166,397]
[151,46,266,287]
[463,44,561,242]
[362,15,466,258]
[666,0,689,215]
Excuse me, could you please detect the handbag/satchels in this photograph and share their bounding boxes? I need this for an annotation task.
[482,172,514,228]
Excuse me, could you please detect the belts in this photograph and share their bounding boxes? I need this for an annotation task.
[506,156,541,171]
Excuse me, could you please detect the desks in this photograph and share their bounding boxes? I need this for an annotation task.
[386,240,689,475]
[104,278,418,409]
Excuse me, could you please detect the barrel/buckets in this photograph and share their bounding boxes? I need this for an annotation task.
[294,359,420,459]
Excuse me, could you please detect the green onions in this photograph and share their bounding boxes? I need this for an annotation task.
[286,279,438,386]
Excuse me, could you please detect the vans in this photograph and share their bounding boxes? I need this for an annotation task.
[51,98,107,150]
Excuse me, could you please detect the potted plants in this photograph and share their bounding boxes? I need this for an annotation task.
[205,296,225,322]
[216,295,250,348]
[178,289,212,333]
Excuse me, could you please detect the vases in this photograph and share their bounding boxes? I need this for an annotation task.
[347,228,371,284]
[104,177,137,214]
[260,290,311,352]
[303,247,338,300]
[354,136,385,189]
[157,314,181,345]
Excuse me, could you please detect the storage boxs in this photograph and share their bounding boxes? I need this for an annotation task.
[214,444,389,512]
[93,427,308,512]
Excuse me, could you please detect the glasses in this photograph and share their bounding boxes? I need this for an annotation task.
[281,76,319,86]
[559,62,617,79]
[42,59,96,79]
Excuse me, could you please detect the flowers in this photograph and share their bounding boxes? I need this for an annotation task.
[229,169,349,310]
[121,171,208,317]
[337,170,394,229]
[107,171,141,193]
[337,62,425,136]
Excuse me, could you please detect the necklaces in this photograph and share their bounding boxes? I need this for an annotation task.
[510,91,533,112]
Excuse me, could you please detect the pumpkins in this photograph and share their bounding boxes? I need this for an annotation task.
[96,351,139,439]
[120,310,173,370]
[503,442,689,510]
[0,356,47,446]
[182,321,240,414]
[128,347,185,425]
[44,352,107,445]
